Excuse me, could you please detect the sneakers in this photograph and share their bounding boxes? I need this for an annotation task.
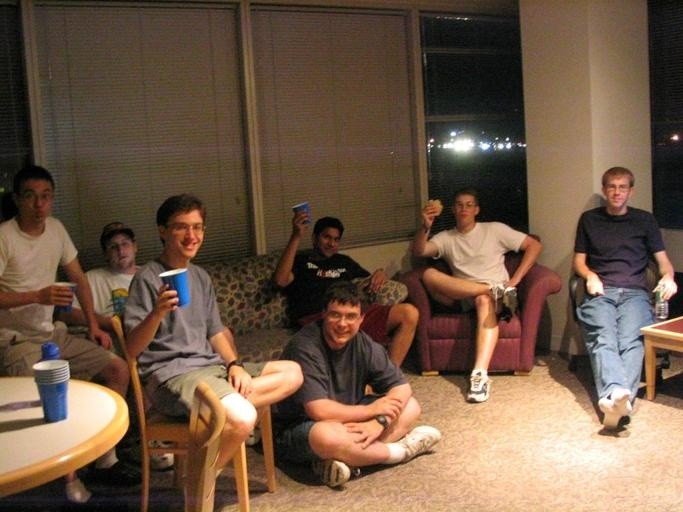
[396,424,442,464]
[130,440,175,471]
[311,456,352,489]
[465,367,493,404]
[491,284,519,313]
[63,491,130,512]
[90,461,143,488]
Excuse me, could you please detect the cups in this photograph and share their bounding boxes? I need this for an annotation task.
[156,268,192,308]
[291,202,309,225]
[53,281,77,314]
[31,359,71,422]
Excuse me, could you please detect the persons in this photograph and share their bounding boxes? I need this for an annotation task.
[121,193,305,486]
[273,209,420,396]
[0,165,144,487]
[411,185,544,404]
[266,278,442,489]
[58,219,174,473]
[570,166,679,433]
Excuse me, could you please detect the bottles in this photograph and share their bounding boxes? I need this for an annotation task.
[39,343,61,360]
[653,289,669,319]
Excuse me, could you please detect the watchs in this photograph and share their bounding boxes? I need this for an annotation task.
[375,414,389,430]
[225,360,244,373]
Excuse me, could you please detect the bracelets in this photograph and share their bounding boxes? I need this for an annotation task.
[421,224,430,234]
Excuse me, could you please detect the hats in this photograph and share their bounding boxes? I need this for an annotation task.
[99,222,135,244]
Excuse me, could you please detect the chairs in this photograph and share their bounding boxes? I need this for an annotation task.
[390,247,560,380]
[563,251,672,398]
[105,311,276,511]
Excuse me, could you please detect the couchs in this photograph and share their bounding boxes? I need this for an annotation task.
[193,245,405,450]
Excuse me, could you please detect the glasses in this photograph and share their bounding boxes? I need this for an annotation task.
[324,310,364,322]
[163,222,208,236]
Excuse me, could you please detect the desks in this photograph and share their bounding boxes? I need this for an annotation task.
[0,372,129,512]
[638,315,683,403]
